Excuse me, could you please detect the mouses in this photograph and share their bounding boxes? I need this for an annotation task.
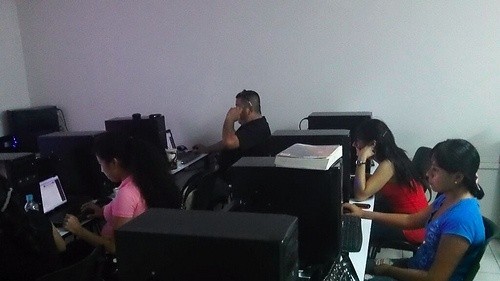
[343,207,351,214]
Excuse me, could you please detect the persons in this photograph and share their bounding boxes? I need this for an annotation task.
[193,89,271,167]
[342,139,486,281]
[352,119,429,243]
[0,203,67,281]
[64,131,148,260]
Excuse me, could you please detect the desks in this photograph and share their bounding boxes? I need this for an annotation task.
[56,148,207,238]
[298,156,379,281]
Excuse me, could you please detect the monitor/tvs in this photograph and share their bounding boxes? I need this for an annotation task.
[166,129,176,149]
[0,134,24,153]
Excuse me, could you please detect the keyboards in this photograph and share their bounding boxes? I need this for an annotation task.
[342,214,363,252]
[181,151,200,164]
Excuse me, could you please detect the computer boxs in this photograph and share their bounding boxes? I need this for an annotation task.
[0,106,372,281]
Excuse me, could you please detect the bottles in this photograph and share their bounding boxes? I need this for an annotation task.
[24,194,39,212]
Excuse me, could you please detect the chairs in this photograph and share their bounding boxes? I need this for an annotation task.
[368,192,445,259]
[450,216,499,281]
[40,172,213,281]
[411,146,435,192]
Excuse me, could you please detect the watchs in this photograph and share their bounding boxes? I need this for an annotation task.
[356,160,367,166]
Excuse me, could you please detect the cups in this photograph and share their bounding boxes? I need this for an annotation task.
[166,148,178,169]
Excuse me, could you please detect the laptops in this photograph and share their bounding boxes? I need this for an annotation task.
[36,175,86,226]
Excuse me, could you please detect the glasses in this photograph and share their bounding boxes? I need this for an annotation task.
[242,89,252,103]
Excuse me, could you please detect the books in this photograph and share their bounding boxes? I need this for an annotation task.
[274,143,342,171]
